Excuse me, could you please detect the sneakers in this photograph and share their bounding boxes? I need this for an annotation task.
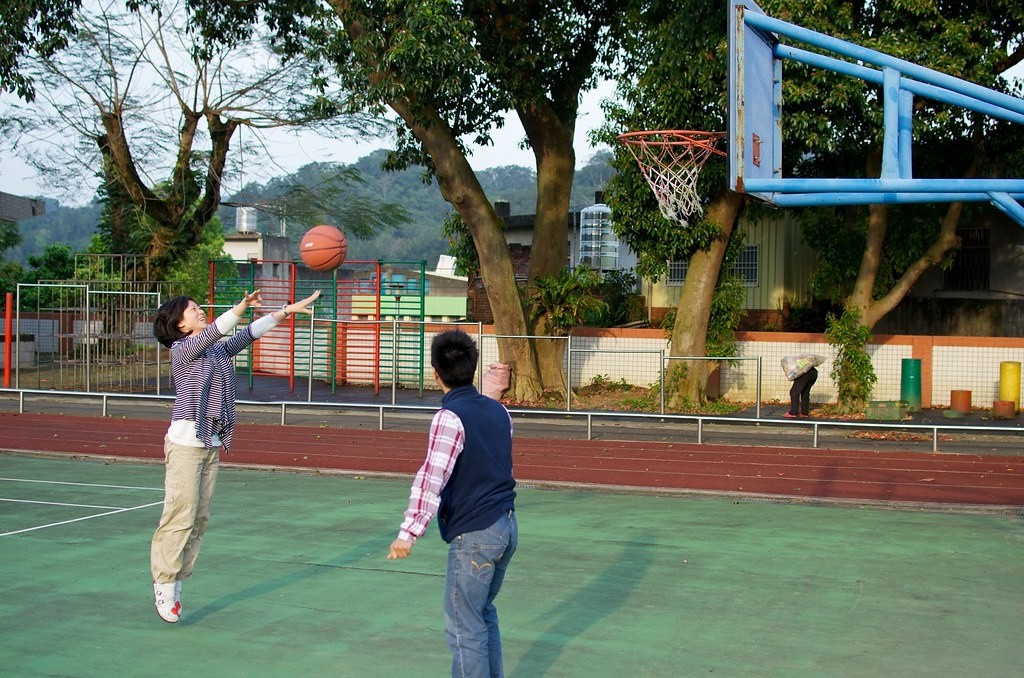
[153,579,183,622]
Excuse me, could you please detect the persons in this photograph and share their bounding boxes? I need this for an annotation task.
[387,329,519,678]
[151,288,322,624]
[783,368,819,418]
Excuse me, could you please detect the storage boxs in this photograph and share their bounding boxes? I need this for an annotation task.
[865,401,909,421]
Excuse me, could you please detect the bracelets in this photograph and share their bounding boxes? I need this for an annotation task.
[281,305,288,318]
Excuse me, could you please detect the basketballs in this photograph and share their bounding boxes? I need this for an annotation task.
[300,224,350,273]
